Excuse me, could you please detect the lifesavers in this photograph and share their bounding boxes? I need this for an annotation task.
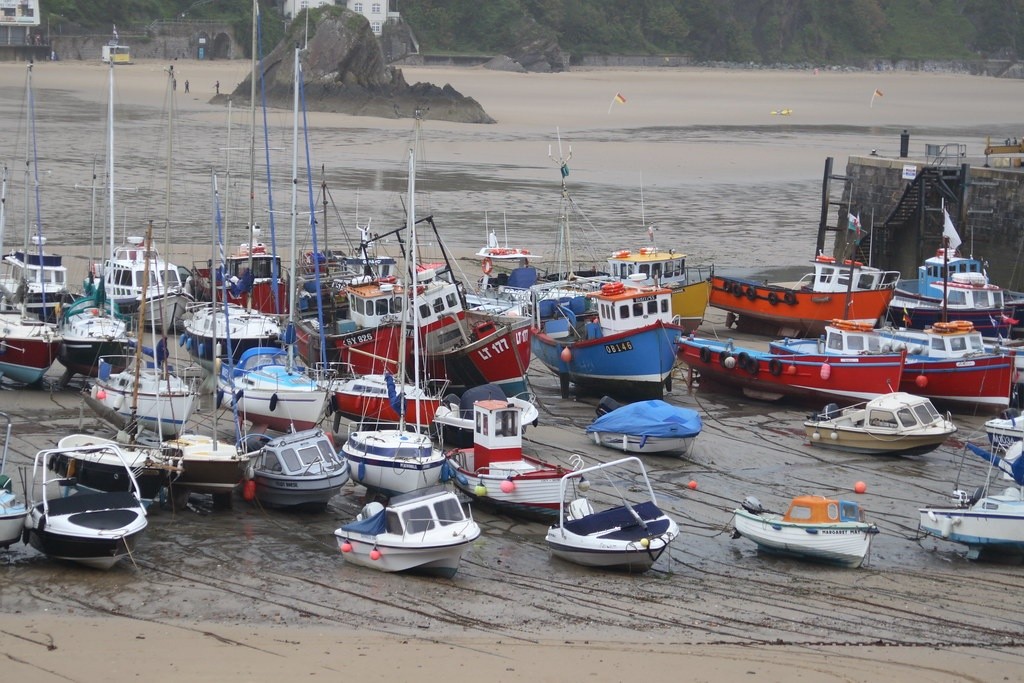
[723,280,732,292]
[931,320,975,334]
[769,358,782,375]
[733,283,741,296]
[746,357,759,373]
[481,257,493,275]
[738,352,750,368]
[374,277,397,285]
[640,247,658,255]
[937,248,956,258]
[602,282,626,296]
[746,286,756,300]
[613,250,632,258]
[831,318,874,332]
[768,291,796,305]
[815,255,862,267]
[252,246,265,253]
[700,347,711,361]
[409,286,424,298]
[720,350,730,367]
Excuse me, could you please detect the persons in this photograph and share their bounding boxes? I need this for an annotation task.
[215,80,219,93]
[51,50,56,61]
[172,79,176,90]
[184,80,189,93]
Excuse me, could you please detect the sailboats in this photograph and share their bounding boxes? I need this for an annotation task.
[0,1,1024,512]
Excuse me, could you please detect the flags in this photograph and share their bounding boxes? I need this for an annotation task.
[113,24,119,39]
[874,91,882,97]
[989,314,1019,328]
[903,308,911,325]
[942,210,961,248]
[615,93,625,104]
[849,214,862,236]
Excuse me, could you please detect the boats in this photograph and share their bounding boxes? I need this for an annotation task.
[913,247,1023,563]
[732,493,881,565]
[801,389,958,456]
[0,410,32,552]
[586,394,704,455]
[21,441,149,573]
[542,455,680,574]
[443,396,583,514]
